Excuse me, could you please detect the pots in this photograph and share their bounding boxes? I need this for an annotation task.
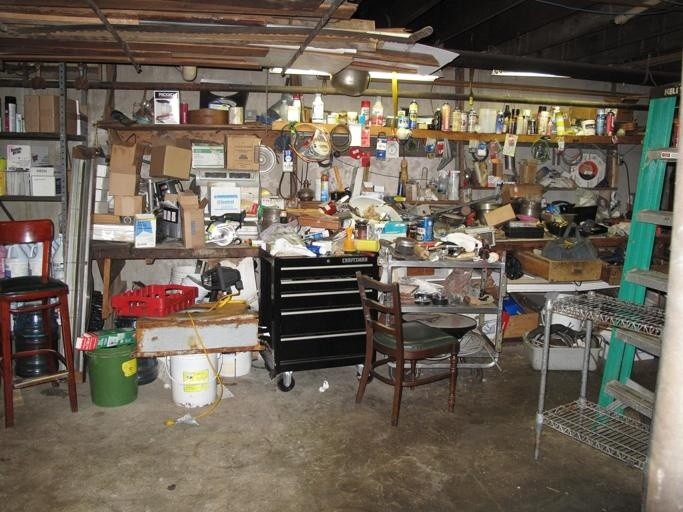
[614,117,639,136]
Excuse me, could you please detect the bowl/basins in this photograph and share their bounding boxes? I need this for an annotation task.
[413,292,447,305]
[189,108,227,124]
[331,191,351,202]
[438,213,466,227]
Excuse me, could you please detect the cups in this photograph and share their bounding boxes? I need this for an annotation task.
[156,98,172,122]
[229,105,244,125]
[395,237,414,256]
[180,102,188,123]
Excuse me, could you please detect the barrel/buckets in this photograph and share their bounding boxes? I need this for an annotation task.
[13,304,60,377]
[166,352,224,408]
[84,343,138,407]
[132,320,159,385]
[220,350,252,377]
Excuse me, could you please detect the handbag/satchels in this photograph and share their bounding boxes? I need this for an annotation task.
[542,224,598,260]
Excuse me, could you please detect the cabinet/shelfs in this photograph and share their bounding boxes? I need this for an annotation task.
[0,61,89,284]
[90,121,262,331]
[265,121,643,254]
[379,251,507,385]
[533,290,664,475]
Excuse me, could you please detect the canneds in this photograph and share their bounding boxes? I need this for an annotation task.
[386,115,410,128]
[417,216,434,241]
[228,107,243,126]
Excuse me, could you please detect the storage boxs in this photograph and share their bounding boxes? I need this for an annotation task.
[90,133,263,252]
[21,94,88,138]
[522,330,604,371]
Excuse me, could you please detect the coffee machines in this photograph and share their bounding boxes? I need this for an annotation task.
[155,179,184,205]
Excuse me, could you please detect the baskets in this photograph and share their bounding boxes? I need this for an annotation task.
[112,285,198,318]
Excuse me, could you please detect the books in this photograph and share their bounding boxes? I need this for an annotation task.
[0,144,56,197]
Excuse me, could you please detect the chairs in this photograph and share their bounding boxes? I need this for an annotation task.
[353,268,461,428]
[0,218,79,428]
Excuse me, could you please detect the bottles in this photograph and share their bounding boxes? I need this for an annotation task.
[486,159,503,182]
[354,222,370,240]
[7,171,30,196]
[303,230,330,255]
[260,206,288,234]
[327,95,615,136]
[139,176,158,211]
[552,145,560,166]
[314,171,328,202]
[253,146,260,163]
[504,154,515,170]
[20,116,24,132]
[397,165,473,202]
[311,94,323,123]
[536,167,550,182]
[280,92,300,122]
[406,215,433,241]
